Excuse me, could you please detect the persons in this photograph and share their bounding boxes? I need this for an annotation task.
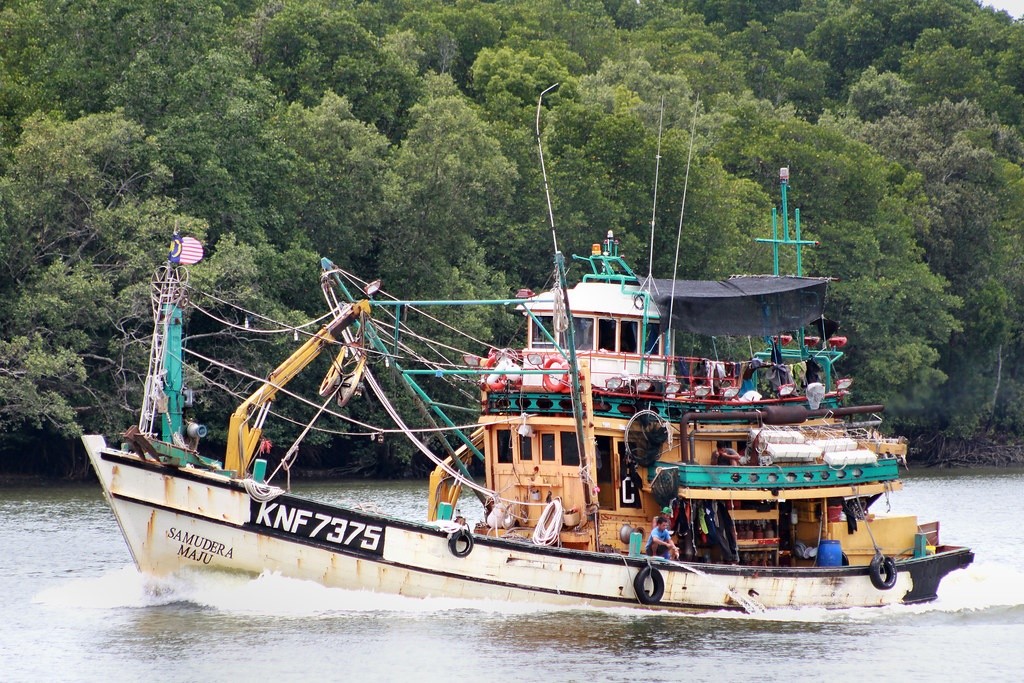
[645,516,680,560]
[711,440,740,466]
[652,507,675,535]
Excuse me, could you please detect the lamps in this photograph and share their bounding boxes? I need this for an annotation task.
[722,385,741,398]
[804,336,820,348]
[665,382,683,394]
[835,378,852,404]
[694,385,712,397]
[769,335,792,346]
[777,383,796,397]
[516,288,535,298]
[822,336,848,349]
[605,376,622,389]
[635,380,653,392]
[526,352,550,369]
[463,354,482,367]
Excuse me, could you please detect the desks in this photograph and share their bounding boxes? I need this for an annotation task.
[740,548,779,567]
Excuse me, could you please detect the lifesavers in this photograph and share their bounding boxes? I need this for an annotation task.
[544,357,569,391]
[448,528,473,558]
[485,355,508,388]
[870,555,896,590]
[634,566,665,605]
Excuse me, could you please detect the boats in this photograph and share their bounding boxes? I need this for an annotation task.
[77,79,976,618]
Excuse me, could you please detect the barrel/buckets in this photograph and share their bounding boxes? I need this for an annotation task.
[827,507,842,523]
[816,540,842,566]
